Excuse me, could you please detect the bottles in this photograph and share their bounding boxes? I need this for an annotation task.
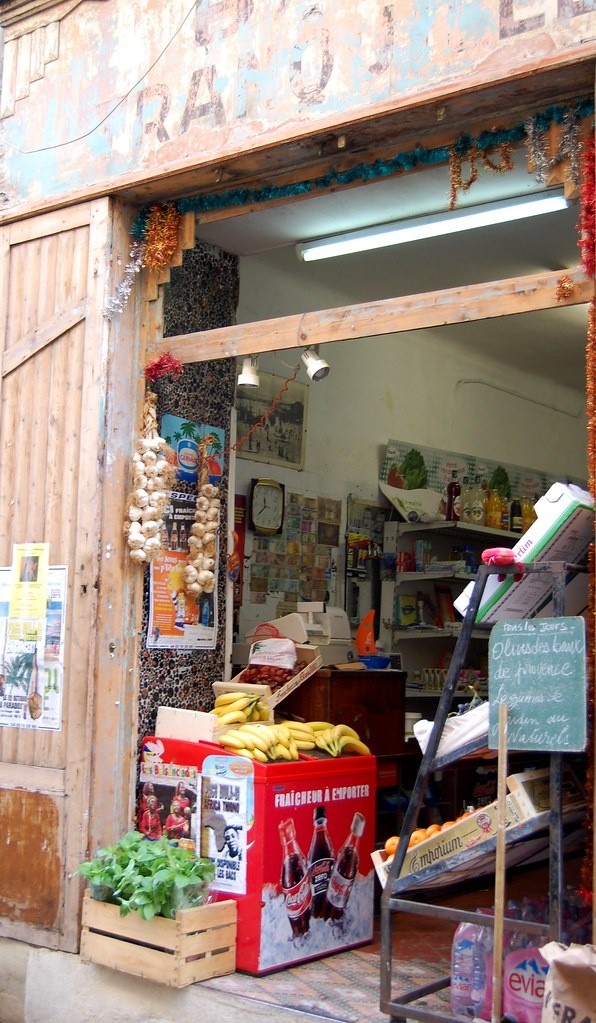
[451,886,592,1023]
[446,470,537,533]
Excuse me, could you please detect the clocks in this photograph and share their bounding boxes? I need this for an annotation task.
[247,477,288,535]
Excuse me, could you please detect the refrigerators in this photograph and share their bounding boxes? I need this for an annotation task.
[138,737,376,977]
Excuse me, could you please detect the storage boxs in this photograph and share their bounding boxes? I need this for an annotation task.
[371,485,593,895]
[154,642,325,743]
[80,889,238,990]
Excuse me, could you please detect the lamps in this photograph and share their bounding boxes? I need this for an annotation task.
[294,187,577,263]
[236,356,261,389]
[300,344,330,382]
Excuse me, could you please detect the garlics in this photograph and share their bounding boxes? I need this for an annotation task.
[183,483,221,597]
[123,435,178,565]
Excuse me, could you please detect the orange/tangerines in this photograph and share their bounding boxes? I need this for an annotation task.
[385,798,496,861]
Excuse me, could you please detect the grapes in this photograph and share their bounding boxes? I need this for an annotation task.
[456,698,487,715]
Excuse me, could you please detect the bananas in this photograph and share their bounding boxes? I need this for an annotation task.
[207,692,371,763]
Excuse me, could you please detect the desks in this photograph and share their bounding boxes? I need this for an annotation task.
[284,668,408,759]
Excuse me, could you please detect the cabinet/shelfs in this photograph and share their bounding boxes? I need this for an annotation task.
[378,519,521,850]
[378,563,575,1023]
